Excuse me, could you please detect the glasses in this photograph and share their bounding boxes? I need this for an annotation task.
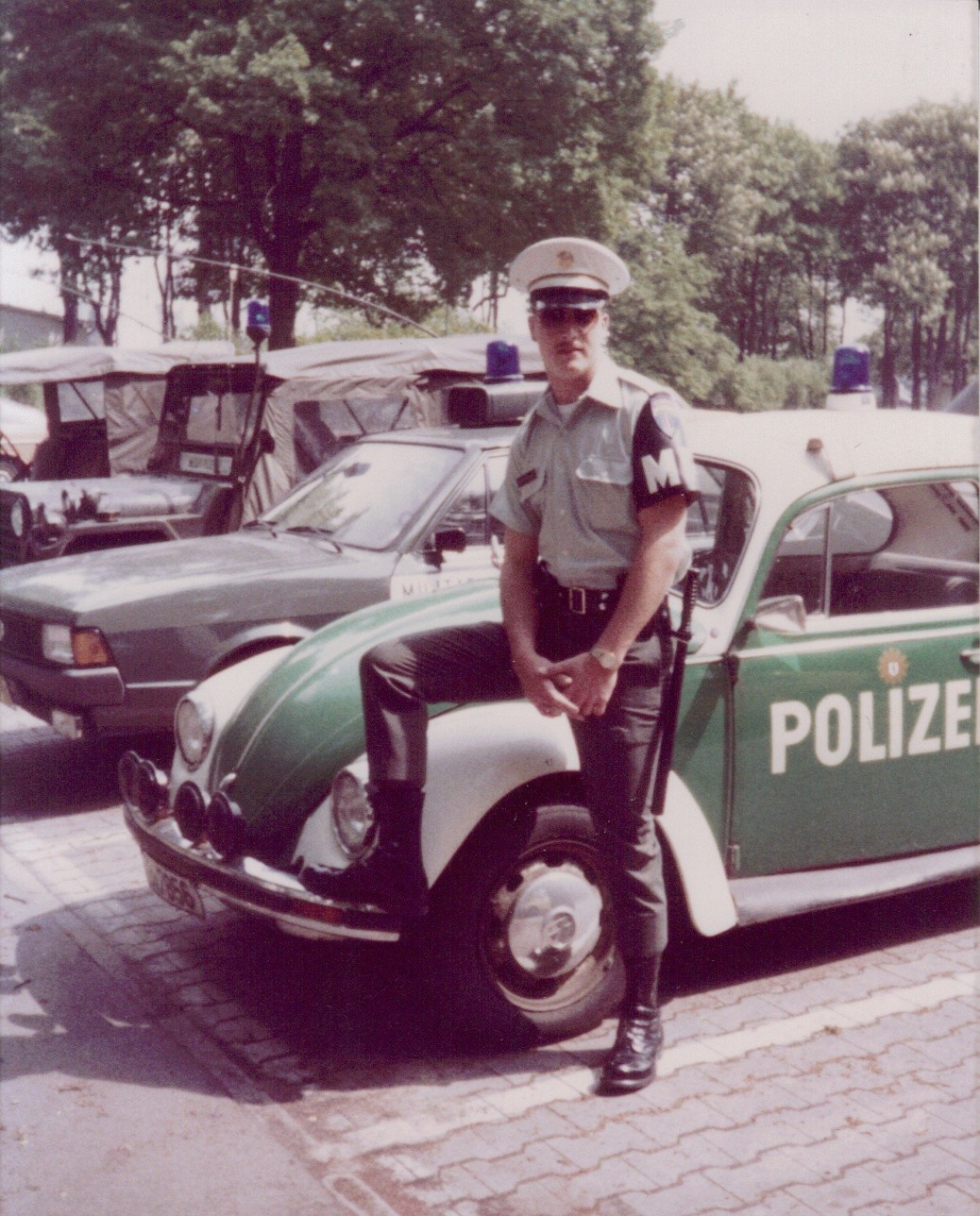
[535,312,599,331]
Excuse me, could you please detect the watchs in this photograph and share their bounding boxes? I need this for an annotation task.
[590,647,622,671]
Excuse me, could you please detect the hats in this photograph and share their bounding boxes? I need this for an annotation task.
[508,234,633,314]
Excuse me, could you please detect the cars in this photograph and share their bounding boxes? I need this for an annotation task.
[0,300,730,737]
[117,343,980,1042]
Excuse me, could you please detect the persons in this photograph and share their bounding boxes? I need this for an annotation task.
[297,237,703,1090]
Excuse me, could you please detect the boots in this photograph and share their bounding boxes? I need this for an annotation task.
[597,961,677,1093]
[293,778,436,918]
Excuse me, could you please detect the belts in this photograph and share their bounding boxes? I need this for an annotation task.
[538,578,629,623]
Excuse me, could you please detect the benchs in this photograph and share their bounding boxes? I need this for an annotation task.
[835,552,980,608]
